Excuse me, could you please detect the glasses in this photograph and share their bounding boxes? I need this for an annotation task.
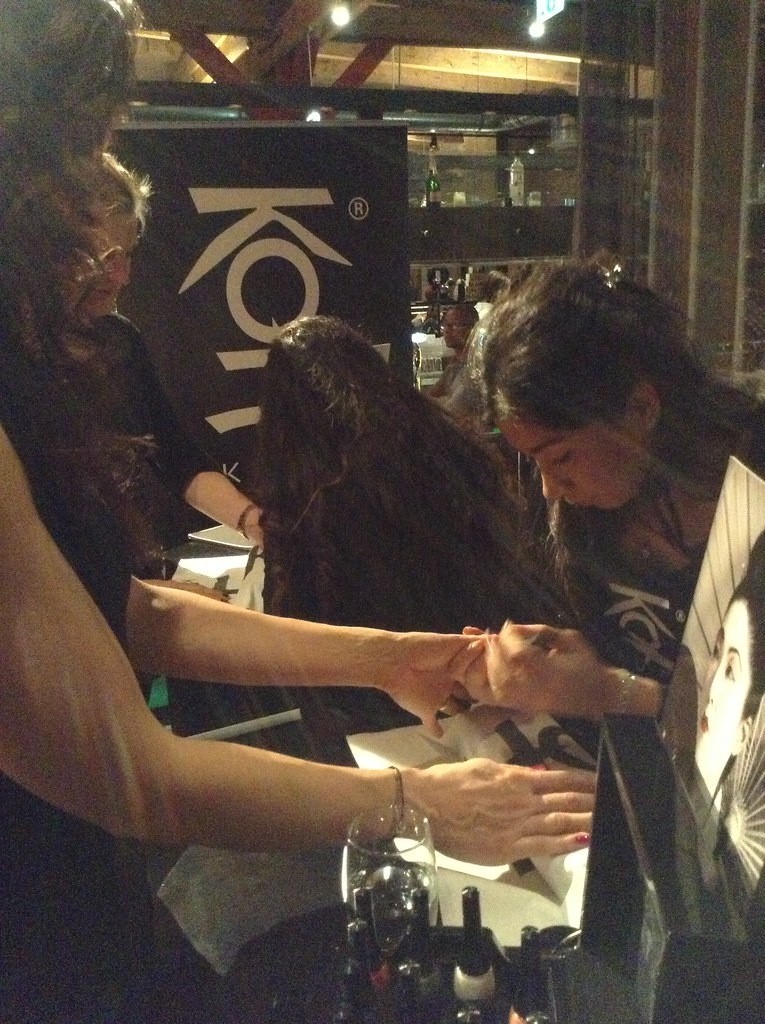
[439,323,472,331]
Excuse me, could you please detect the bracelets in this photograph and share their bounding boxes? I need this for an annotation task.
[614,667,634,715]
[383,767,404,846]
[235,503,259,540]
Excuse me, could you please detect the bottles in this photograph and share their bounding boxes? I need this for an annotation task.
[508,153,524,206]
[424,147,440,207]
[330,883,555,1023]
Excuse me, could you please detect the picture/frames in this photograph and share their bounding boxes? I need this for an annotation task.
[656,455,764,919]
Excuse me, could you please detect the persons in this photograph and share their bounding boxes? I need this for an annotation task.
[408,225,543,431]
[2,1,598,994]
[256,315,578,773]
[445,252,764,732]
[67,150,271,612]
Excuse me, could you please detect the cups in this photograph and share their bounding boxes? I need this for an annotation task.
[342,804,439,945]
